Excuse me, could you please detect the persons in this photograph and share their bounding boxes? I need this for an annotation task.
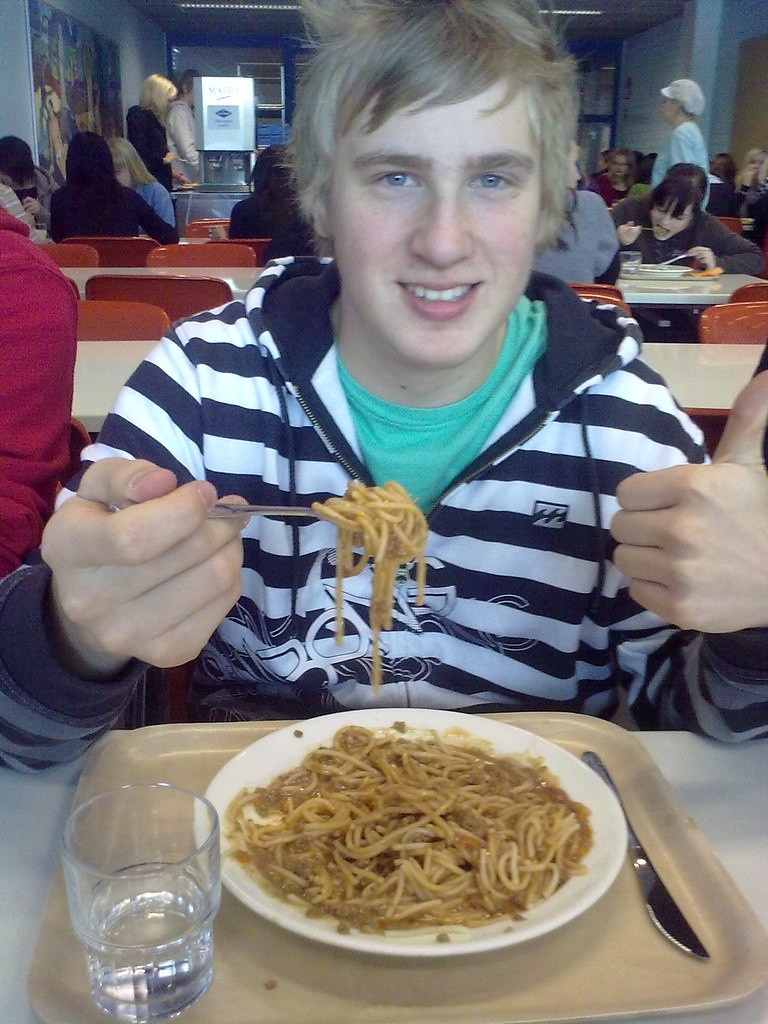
[0,68,335,267]
[0,206,79,581]
[650,81,711,213]
[0,0,768,775]
[534,145,768,348]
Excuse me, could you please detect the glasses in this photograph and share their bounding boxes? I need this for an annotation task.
[614,161,629,166]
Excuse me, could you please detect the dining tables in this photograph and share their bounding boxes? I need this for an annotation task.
[0,727,768,1024]
[61,337,765,432]
[59,264,768,307]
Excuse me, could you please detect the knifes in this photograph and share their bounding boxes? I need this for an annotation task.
[582,751,709,959]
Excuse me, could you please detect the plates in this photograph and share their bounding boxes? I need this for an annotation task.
[179,184,200,187]
[205,707,627,957]
[639,264,694,278]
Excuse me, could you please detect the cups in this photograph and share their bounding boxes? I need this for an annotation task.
[619,252,642,277]
[62,783,221,1022]
[34,223,46,241]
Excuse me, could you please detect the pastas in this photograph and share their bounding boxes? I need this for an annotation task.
[313,476,428,691]
[220,727,592,931]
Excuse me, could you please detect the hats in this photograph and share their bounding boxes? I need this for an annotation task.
[660,79,704,115]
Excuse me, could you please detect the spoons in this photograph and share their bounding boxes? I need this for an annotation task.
[629,226,666,234]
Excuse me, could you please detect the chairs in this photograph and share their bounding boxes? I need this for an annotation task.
[23,219,768,496]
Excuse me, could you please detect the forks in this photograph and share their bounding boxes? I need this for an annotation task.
[108,493,419,517]
